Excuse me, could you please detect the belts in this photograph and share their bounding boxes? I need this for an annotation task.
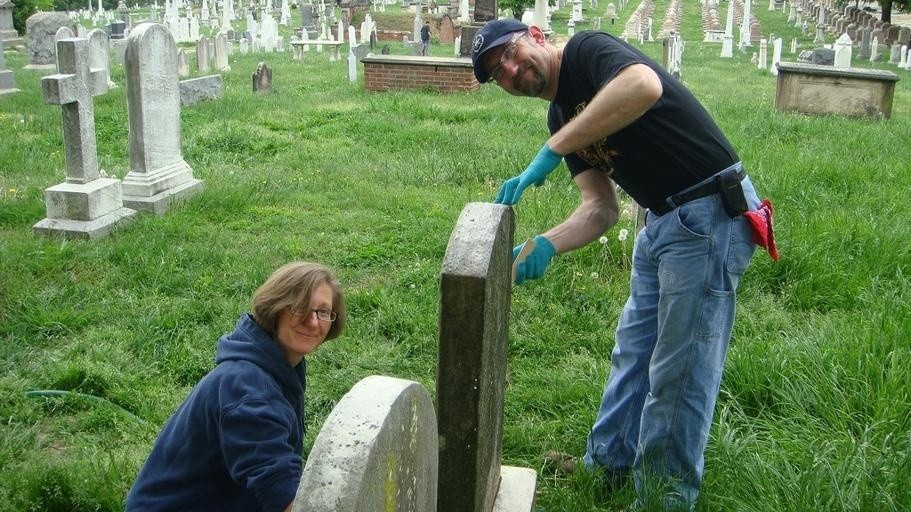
[648,169,747,218]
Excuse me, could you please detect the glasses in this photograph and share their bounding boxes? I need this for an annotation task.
[287,304,337,323]
[488,30,528,83]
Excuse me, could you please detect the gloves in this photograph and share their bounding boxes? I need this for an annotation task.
[513,235,557,286]
[493,141,563,206]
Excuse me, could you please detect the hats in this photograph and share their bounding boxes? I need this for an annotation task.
[470,18,529,85]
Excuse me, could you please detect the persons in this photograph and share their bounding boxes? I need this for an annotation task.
[470,18,781,511]
[420,21,433,54]
[124,260,347,511]
